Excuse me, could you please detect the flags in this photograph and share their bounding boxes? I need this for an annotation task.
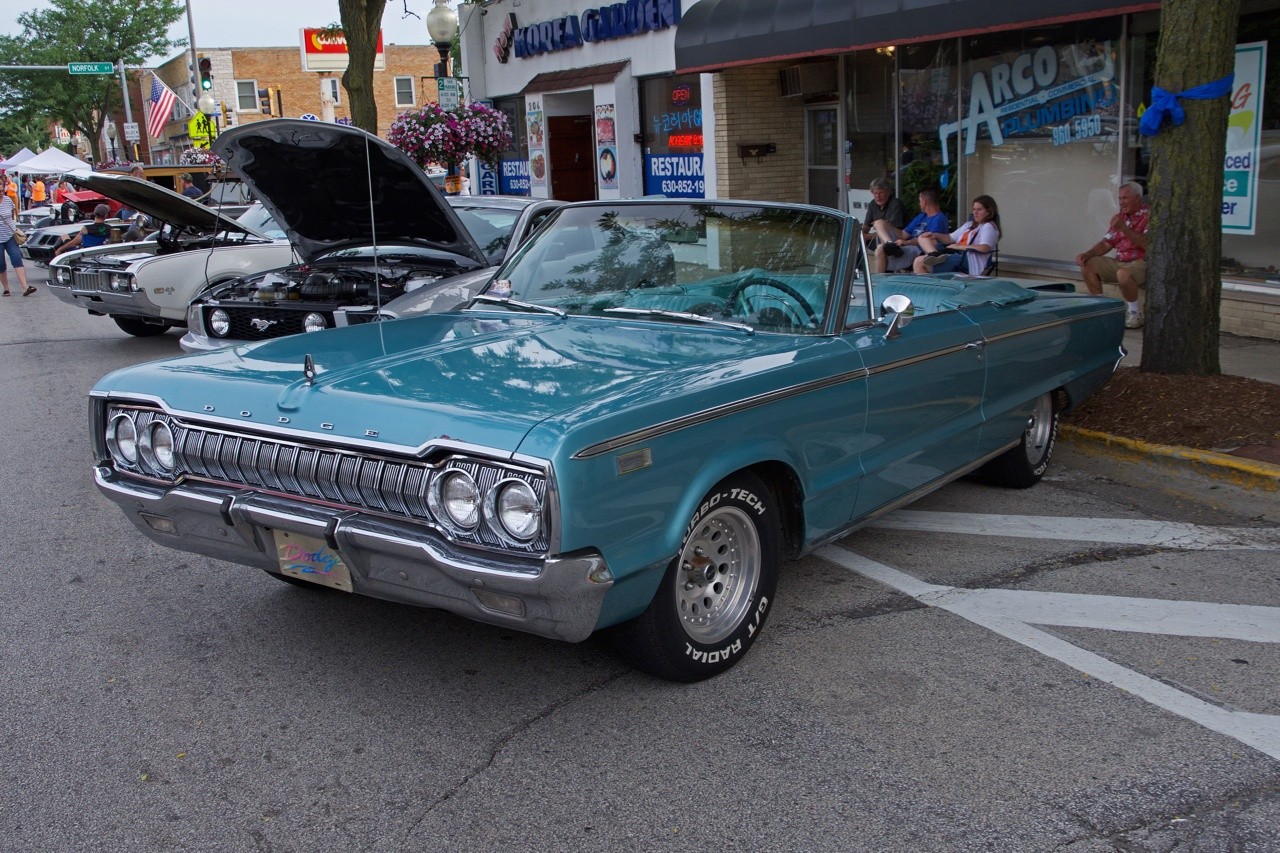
[146,76,176,138]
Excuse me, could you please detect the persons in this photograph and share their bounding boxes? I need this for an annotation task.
[179,173,203,199]
[0,175,153,297]
[874,188,948,273]
[856,177,909,270]
[1077,181,1148,329]
[913,195,1003,275]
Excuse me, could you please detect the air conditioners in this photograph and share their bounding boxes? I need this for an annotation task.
[779,60,837,98]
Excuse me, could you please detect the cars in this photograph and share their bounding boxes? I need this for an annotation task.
[16,187,181,268]
[44,168,304,338]
[176,115,677,358]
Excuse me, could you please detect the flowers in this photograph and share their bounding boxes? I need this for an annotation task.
[94,161,130,170]
[387,100,515,171]
[179,148,224,177]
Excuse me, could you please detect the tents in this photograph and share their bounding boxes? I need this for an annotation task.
[0,146,93,210]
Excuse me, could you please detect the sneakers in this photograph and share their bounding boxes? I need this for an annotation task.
[1124,312,1144,329]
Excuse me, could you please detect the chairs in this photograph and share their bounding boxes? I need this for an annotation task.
[65,234,109,252]
[958,241,999,277]
[743,272,829,323]
[624,294,726,315]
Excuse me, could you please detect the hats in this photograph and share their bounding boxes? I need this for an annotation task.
[96,203,110,215]
[178,173,192,182]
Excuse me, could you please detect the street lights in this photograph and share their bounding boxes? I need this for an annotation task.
[427,1,459,196]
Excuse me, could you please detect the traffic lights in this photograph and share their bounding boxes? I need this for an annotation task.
[189,53,198,109]
[198,58,212,90]
[222,101,233,128]
[258,87,275,116]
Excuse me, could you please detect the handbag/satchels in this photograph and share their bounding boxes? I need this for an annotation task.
[14,228,27,244]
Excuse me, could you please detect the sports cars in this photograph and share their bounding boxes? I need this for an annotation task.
[76,189,1133,681]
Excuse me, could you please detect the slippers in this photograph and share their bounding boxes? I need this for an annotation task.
[23,286,37,296]
[3,290,10,296]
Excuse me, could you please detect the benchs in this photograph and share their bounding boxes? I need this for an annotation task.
[851,274,965,324]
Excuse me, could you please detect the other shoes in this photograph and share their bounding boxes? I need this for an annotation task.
[924,252,948,265]
[883,243,905,258]
